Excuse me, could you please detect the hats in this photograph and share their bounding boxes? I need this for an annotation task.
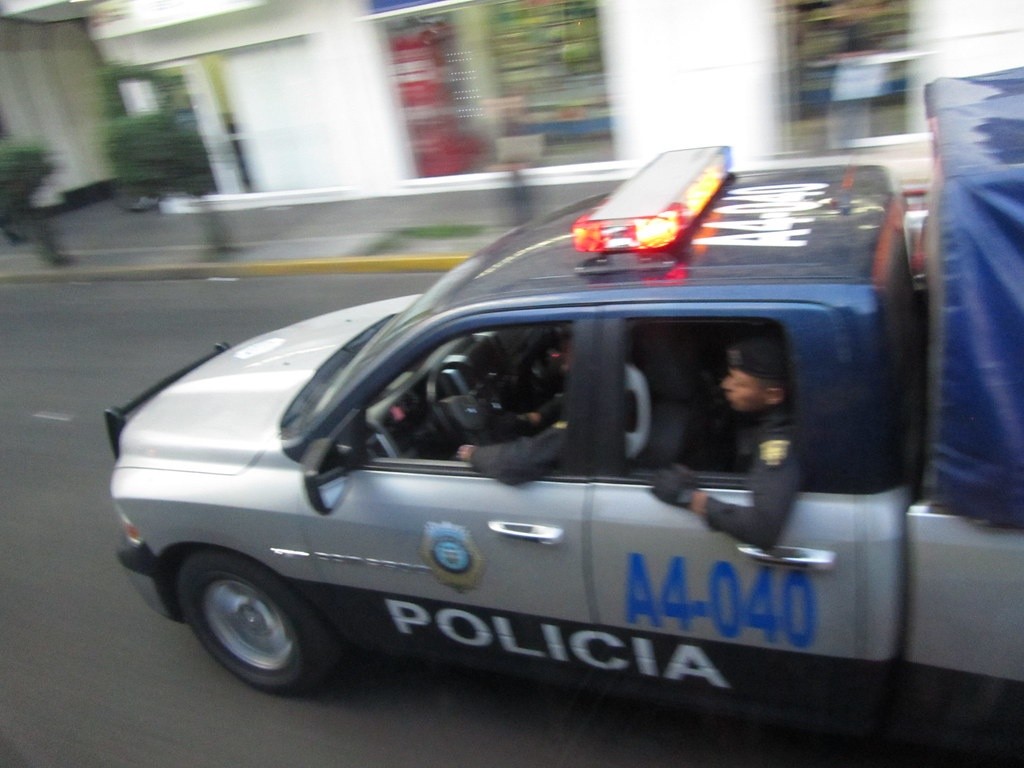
[728,338,790,380]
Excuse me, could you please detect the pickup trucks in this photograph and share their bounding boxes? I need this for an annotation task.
[100,69,1024,767]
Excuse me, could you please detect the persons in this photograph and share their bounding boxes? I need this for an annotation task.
[455,332,571,487]
[647,338,803,549]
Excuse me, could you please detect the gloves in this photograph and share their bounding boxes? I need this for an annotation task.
[649,464,697,508]
